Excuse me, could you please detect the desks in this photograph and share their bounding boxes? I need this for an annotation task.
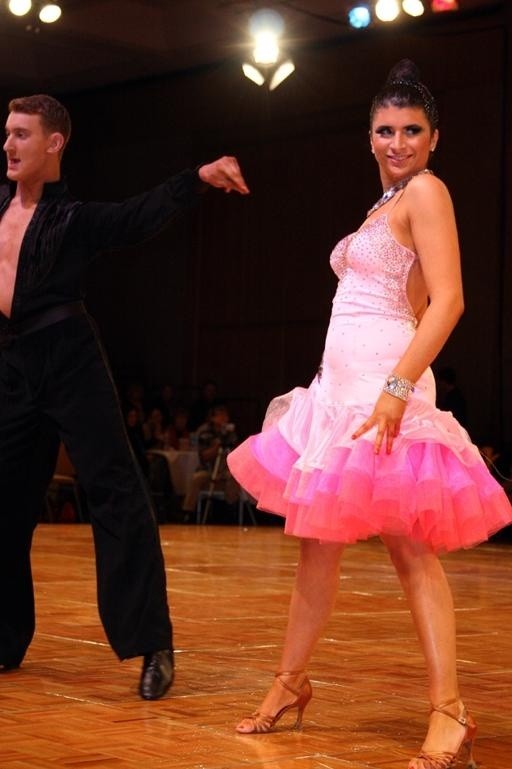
[154,448,197,496]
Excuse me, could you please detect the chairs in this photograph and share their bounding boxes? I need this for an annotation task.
[190,446,257,525]
[143,451,187,523]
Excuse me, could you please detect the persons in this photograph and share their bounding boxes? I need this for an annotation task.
[226,62,512,769]
[44,397,264,522]
[480,443,509,492]
[0,96,255,702]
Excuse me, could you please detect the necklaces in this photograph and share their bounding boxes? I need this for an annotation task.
[366,167,431,220]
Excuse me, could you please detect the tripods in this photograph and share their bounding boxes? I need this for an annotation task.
[203,446,256,525]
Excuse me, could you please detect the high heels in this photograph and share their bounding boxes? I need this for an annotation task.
[407,693,478,769]
[235,668,312,733]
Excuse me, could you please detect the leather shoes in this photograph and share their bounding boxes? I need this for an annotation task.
[138,645,174,699]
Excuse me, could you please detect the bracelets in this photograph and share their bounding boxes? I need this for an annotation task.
[381,376,417,403]
